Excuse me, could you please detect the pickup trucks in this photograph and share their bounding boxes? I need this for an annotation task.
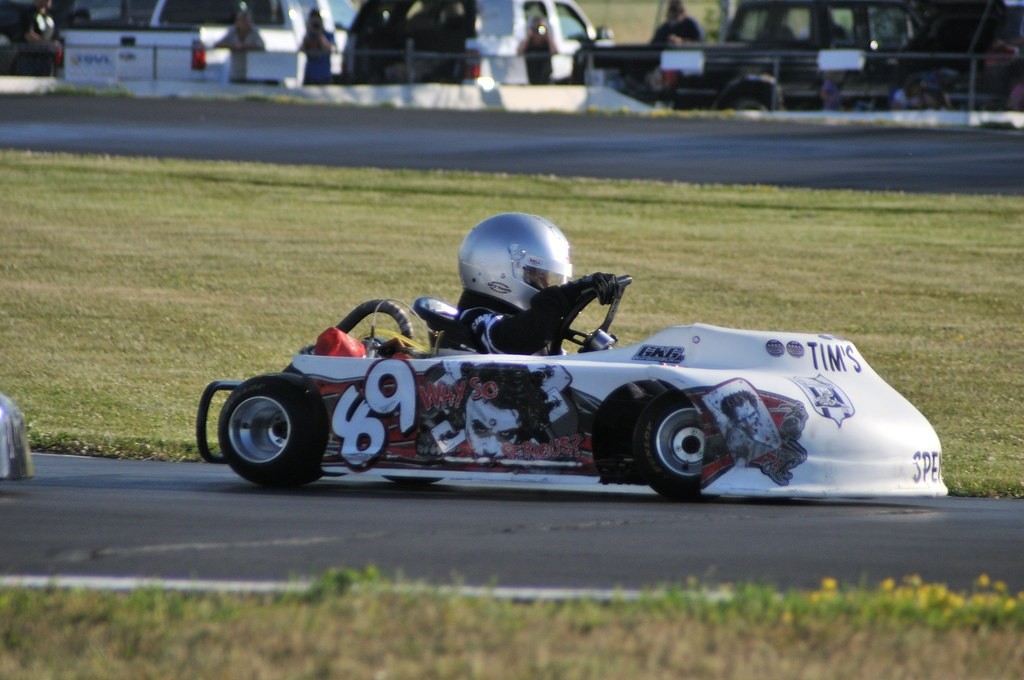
[61,2,347,87]
[573,0,926,113]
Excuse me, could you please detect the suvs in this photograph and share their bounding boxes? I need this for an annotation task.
[342,0,615,84]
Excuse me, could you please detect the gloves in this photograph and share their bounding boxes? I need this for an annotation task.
[560,272,622,306]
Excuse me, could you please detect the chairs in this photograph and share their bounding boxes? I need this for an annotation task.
[411,297,474,359]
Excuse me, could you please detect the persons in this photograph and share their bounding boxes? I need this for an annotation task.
[213,8,266,83]
[650,0,705,46]
[299,10,338,85]
[10,0,61,74]
[890,73,946,111]
[445,212,620,355]
[820,70,846,112]
[516,16,558,85]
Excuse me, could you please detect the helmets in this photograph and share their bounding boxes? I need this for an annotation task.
[458,213,572,316]
